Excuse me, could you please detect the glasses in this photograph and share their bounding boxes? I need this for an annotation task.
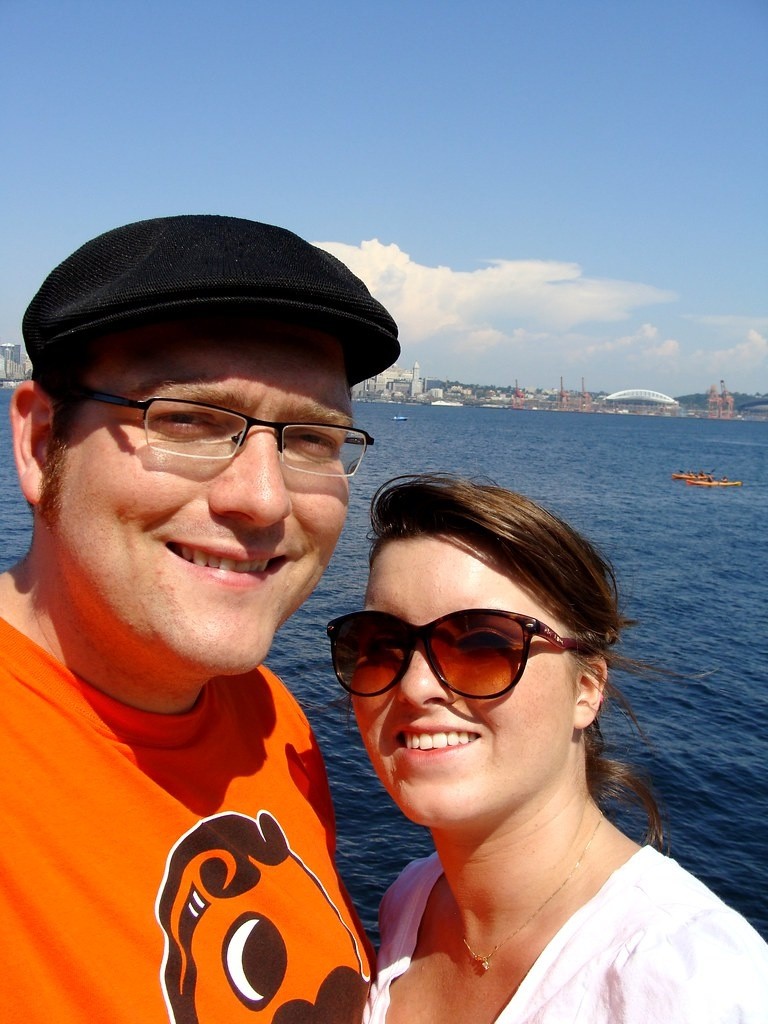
[327,609,577,699]
[67,383,375,477]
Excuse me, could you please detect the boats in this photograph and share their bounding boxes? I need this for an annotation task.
[685,479,743,486]
[390,412,408,421]
[672,471,715,480]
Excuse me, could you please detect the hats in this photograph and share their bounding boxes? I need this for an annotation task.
[23,215,401,389]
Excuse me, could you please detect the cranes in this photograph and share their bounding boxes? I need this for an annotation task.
[511,379,525,409]
[706,380,734,420]
[577,376,591,411]
[558,376,572,408]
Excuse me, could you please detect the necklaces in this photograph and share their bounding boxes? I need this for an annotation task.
[458,811,602,972]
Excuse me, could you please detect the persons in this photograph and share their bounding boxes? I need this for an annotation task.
[328,475,768,1024]
[0,214,402,1024]
